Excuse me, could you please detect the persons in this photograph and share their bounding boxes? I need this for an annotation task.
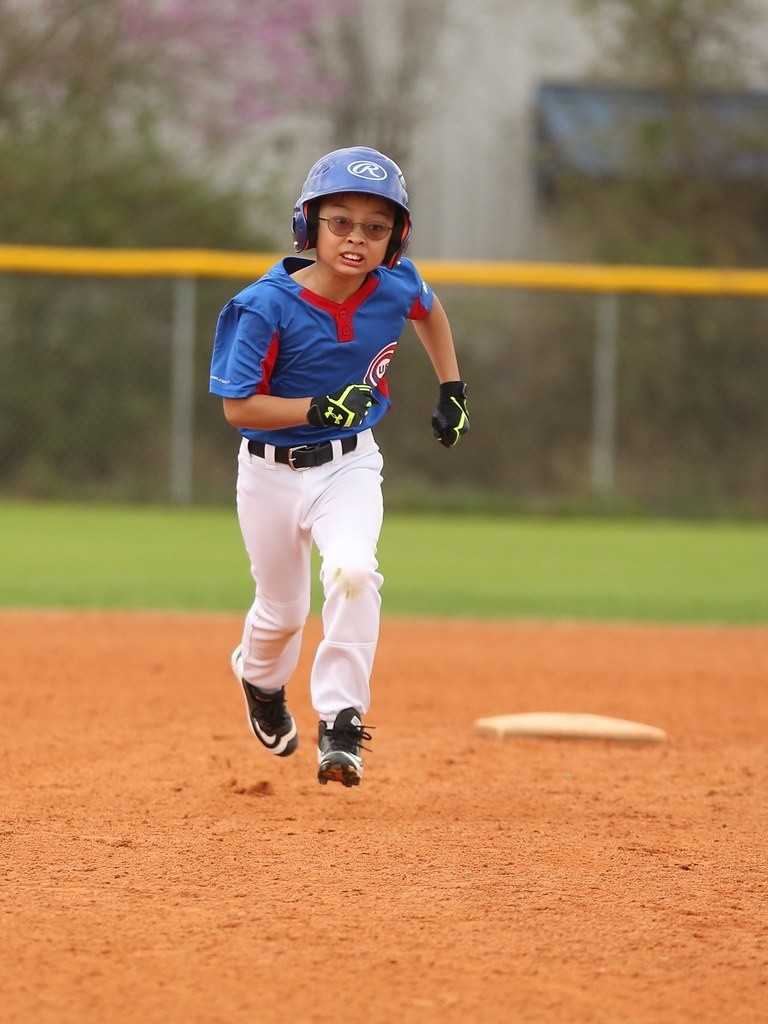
[208,145,470,787]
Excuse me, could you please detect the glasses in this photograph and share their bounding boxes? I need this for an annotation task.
[315,216,394,240]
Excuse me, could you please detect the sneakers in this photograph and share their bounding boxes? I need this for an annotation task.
[317,706,375,786]
[232,646,298,756]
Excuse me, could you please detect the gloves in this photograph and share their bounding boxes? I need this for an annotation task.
[431,381,469,448]
[307,384,373,428]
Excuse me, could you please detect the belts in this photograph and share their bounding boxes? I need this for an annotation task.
[249,435,358,471]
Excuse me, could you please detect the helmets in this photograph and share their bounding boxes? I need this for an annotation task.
[293,146,413,271]
